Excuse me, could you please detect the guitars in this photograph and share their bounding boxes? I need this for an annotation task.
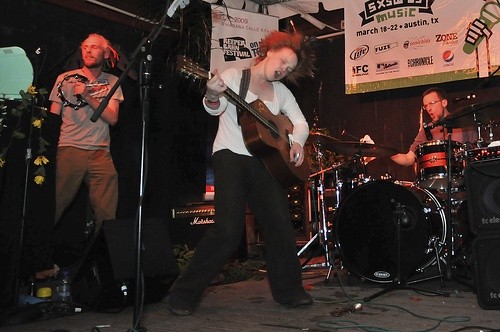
[175,54,311,186]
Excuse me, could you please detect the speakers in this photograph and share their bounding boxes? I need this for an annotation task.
[472,234,500,310]
[68,203,260,311]
[466,158,500,234]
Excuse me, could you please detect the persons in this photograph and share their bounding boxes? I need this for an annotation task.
[168,27,312,316]
[359,87,478,166]
[36,34,125,279]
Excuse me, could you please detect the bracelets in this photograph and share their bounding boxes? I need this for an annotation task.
[206,95,219,103]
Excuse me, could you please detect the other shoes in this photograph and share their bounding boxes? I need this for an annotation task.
[278,289,314,307]
[166,300,198,317]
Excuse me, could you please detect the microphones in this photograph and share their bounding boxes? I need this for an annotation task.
[423,121,433,141]
[400,213,411,228]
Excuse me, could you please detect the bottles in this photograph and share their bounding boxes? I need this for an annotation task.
[58,271,72,315]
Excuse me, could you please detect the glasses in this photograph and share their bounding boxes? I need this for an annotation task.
[422,100,441,110]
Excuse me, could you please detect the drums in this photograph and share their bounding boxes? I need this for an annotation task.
[415,140,464,190]
[333,179,447,283]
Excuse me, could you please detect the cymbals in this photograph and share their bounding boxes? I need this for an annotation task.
[56,73,91,111]
[308,130,400,158]
[440,99,500,129]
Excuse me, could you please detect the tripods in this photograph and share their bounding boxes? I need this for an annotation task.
[297,99,500,311]
